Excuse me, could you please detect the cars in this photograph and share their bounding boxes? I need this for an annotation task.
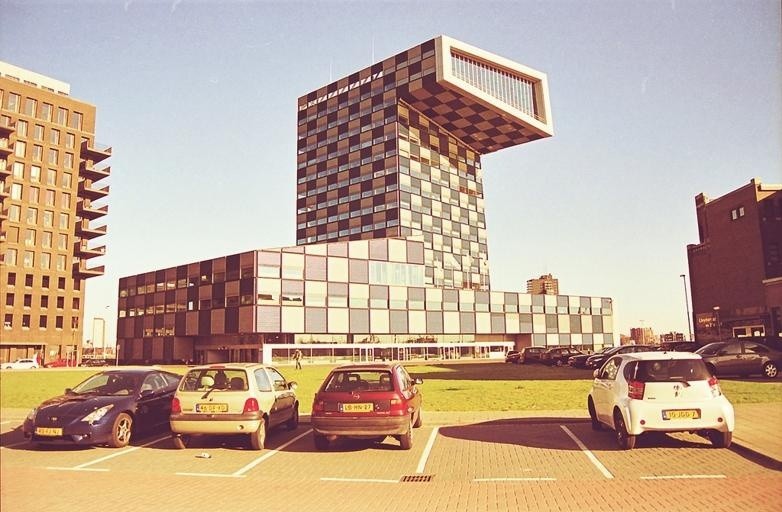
[309,360,425,451]
[168,360,301,451]
[502,338,782,380]
[22,367,184,448]
[0,356,112,368]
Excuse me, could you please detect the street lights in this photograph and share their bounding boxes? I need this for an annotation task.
[679,273,692,342]
[712,305,722,342]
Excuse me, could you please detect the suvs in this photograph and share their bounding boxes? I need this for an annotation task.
[586,348,738,451]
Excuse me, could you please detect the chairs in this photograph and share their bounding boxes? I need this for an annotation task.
[344,374,390,390]
[197,373,244,391]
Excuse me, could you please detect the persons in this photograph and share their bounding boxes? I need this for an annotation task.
[292,348,304,371]
